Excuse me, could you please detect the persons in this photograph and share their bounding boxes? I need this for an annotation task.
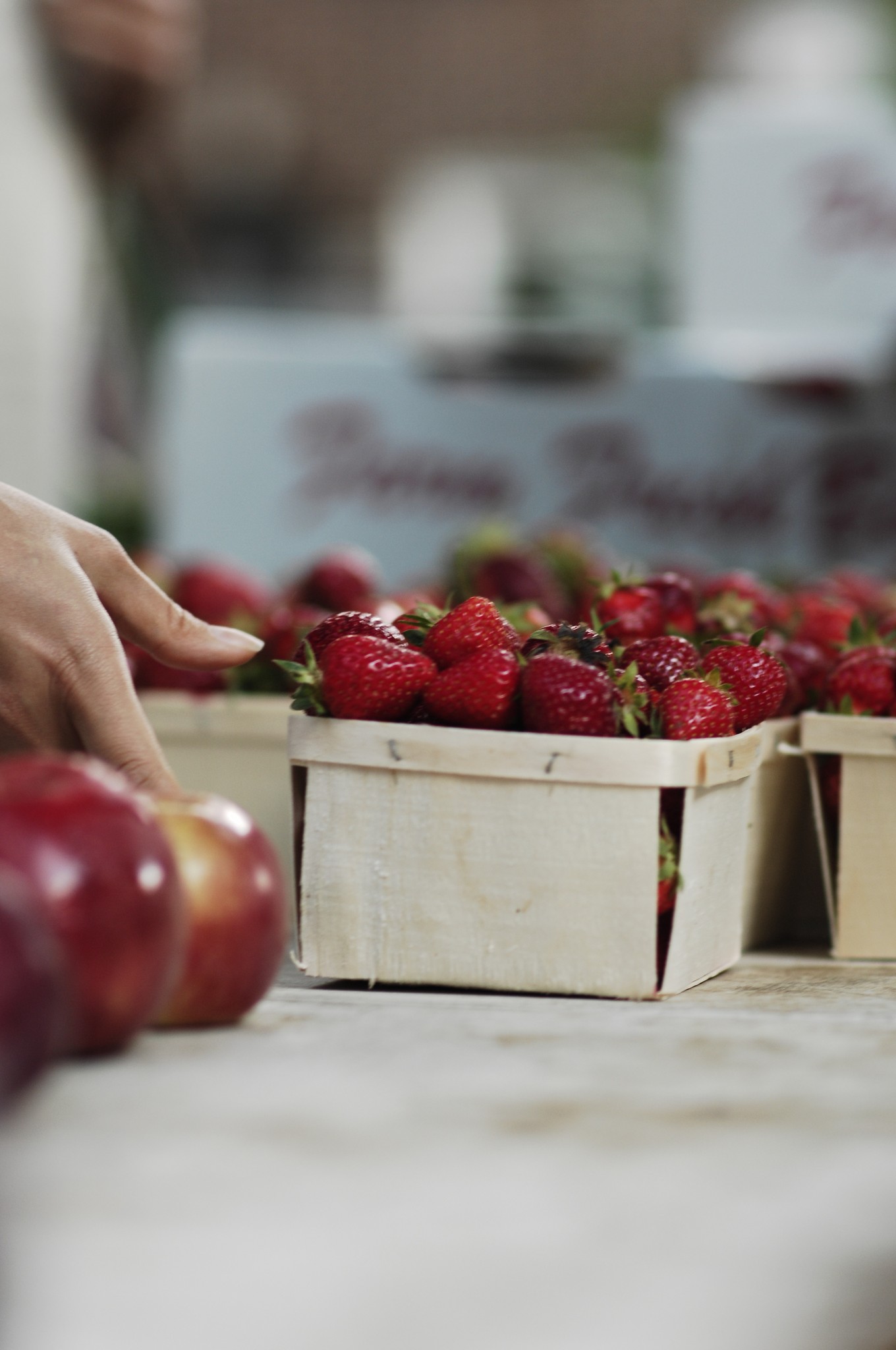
[0,478,266,796]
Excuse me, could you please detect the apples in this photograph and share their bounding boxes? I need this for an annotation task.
[0,748,286,1114]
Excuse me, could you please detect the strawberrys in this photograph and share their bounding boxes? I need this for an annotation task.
[86,496,895,921]
